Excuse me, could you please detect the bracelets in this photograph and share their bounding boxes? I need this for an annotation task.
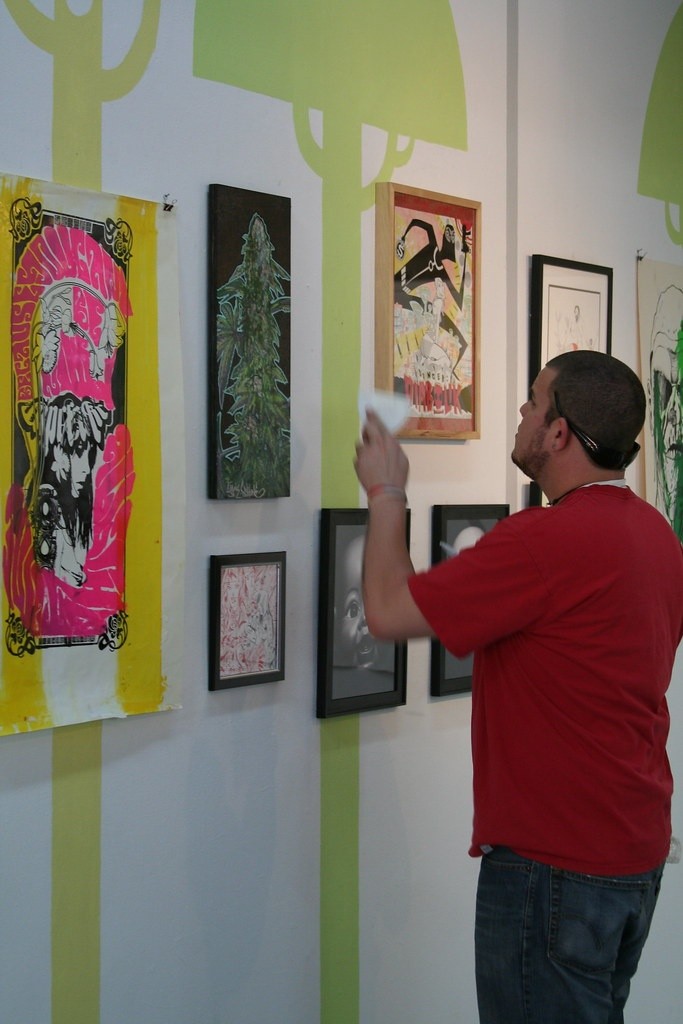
[367,483,407,500]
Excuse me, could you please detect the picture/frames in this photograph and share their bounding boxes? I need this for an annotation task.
[374,182,484,441]
[207,551,288,690]
[430,503,510,698]
[315,507,411,719]
[526,253,615,407]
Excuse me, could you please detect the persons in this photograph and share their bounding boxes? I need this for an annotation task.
[354,348,683,1023]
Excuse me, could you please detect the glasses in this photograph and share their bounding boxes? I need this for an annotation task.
[552,390,641,470]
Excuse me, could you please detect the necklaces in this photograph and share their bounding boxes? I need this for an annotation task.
[545,481,589,507]
